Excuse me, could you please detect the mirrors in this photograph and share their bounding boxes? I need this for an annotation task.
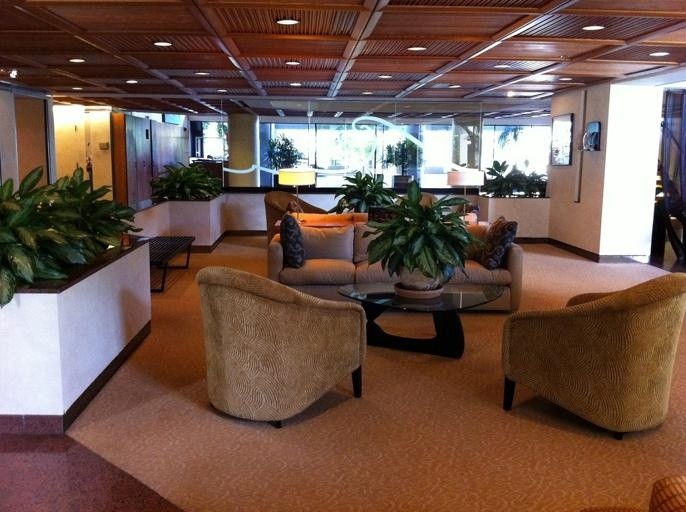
[453,123,481,171]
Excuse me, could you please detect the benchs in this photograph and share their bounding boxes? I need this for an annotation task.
[140,236,195,291]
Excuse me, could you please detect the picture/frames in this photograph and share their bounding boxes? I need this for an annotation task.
[550,112,574,166]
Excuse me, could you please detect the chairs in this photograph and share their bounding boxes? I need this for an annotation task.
[263,190,328,247]
[395,191,441,215]
[502,266,686,439]
[195,265,369,426]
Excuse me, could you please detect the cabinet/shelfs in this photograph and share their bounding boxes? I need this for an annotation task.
[112,112,154,212]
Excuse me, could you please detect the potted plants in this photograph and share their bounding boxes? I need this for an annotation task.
[383,138,423,192]
[351,178,488,298]
[264,131,303,188]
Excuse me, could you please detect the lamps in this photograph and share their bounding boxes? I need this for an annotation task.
[446,170,484,222]
[279,169,315,214]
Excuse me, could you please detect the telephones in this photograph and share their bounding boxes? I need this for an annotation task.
[583,131,598,151]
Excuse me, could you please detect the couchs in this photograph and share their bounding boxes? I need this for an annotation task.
[267,222,525,312]
[276,210,478,228]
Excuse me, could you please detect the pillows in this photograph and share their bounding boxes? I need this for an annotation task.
[287,198,303,213]
[475,215,518,269]
[456,201,481,218]
[280,213,303,268]
